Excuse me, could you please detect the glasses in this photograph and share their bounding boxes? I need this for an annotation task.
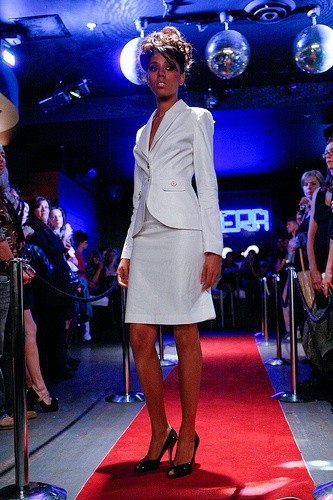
[322,151,333,158]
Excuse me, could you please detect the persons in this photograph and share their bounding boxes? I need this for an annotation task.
[0,143,120,430]
[216,137,333,413]
[116,27,224,479]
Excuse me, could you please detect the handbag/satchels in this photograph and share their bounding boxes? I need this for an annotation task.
[301,286,333,371]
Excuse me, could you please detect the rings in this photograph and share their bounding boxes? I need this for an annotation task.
[322,285,325,287]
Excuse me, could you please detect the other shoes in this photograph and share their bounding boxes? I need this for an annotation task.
[10,410,38,420]
[0,414,30,430]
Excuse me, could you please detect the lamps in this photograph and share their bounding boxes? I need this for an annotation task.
[38,91,71,117]
[78,79,91,97]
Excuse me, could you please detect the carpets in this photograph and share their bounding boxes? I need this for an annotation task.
[73,335,318,500]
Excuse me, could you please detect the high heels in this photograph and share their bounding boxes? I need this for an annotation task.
[134,426,178,473]
[166,432,200,479]
[27,385,59,412]
